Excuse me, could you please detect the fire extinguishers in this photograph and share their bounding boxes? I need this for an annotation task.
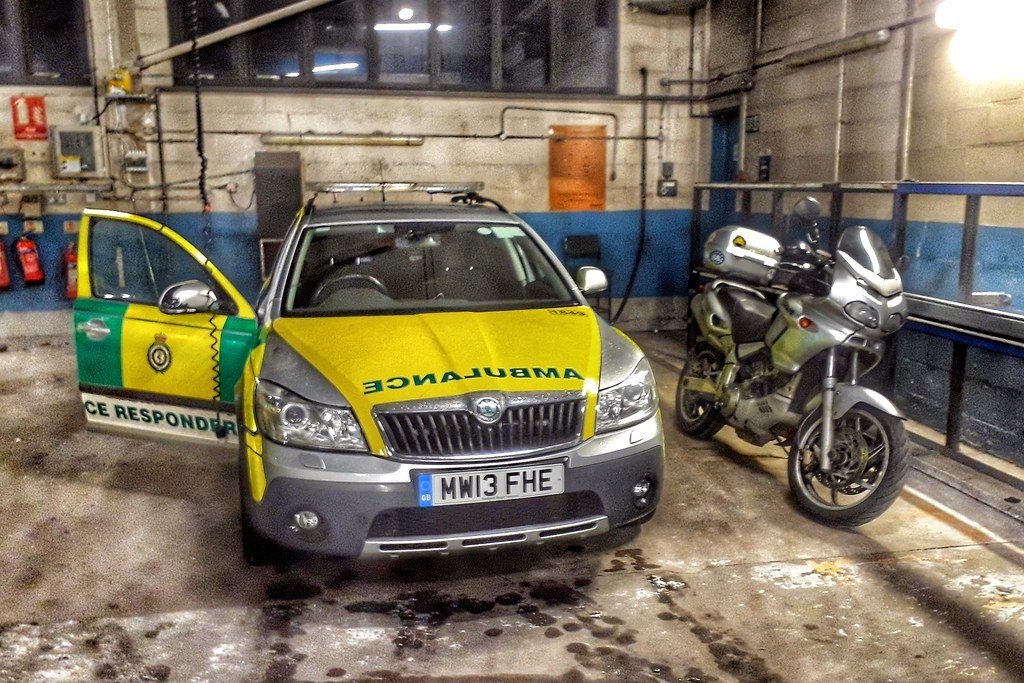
[62,241,78,298]
[11,229,48,287]
[0,237,11,289]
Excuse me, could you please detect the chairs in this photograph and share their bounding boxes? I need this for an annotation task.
[563,234,614,320]
[316,237,387,304]
[423,239,500,301]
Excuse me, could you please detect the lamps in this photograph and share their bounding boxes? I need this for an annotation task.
[782,28,892,67]
[259,134,424,146]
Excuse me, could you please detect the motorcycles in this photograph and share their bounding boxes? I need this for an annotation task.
[673,196,911,530]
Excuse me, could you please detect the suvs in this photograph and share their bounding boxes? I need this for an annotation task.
[69,179,664,567]
